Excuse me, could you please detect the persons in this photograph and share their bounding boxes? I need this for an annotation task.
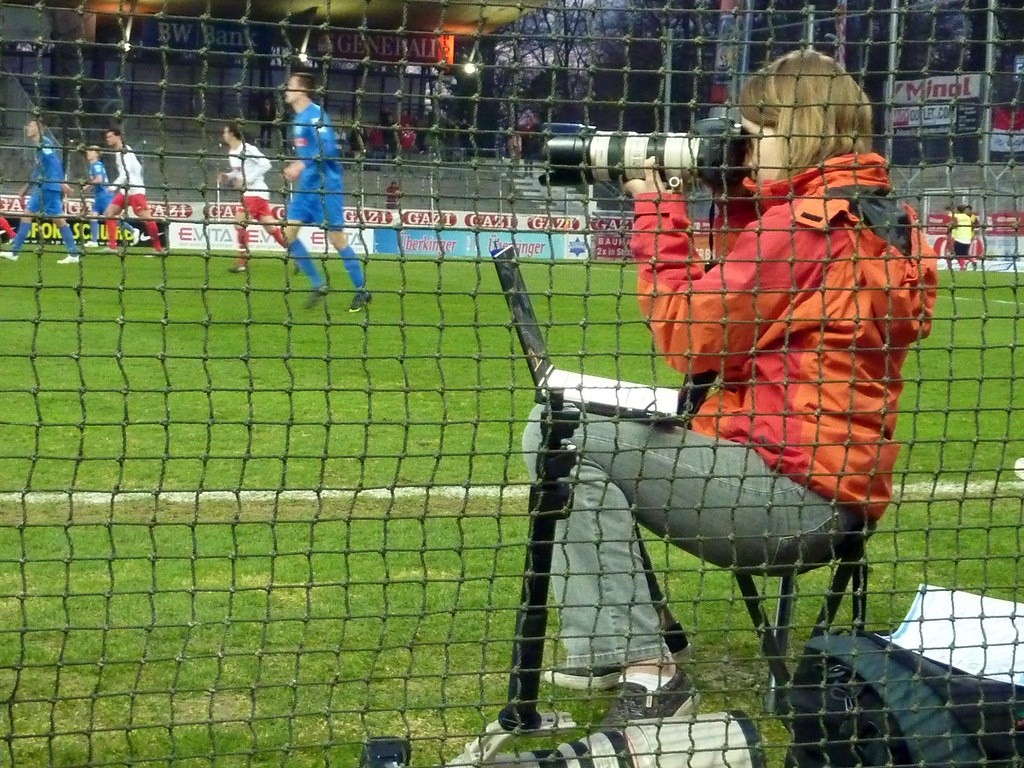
[942,204,996,273]
[386,180,403,209]
[81,142,114,248]
[521,50,938,732]
[0,215,19,242]
[282,70,373,313]
[0,116,80,264]
[216,117,287,272]
[259,95,275,149]
[337,118,418,158]
[100,129,163,254]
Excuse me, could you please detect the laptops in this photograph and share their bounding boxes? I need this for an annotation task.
[493,246,687,425]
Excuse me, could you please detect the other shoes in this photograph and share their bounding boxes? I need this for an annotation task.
[96,248,118,254]
[228,266,246,273]
[293,260,301,275]
[306,292,323,308]
[84,241,98,248]
[345,290,371,312]
[0,251,19,261]
[57,255,79,263]
[144,251,164,257]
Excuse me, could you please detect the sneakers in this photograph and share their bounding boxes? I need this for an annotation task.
[545,621,691,690]
[594,663,700,734]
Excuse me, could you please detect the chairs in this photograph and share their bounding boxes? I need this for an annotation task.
[636,369,868,720]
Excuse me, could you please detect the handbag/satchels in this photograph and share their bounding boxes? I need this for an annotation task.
[784,629,1024,768]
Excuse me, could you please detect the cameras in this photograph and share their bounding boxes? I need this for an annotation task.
[539,116,747,186]
